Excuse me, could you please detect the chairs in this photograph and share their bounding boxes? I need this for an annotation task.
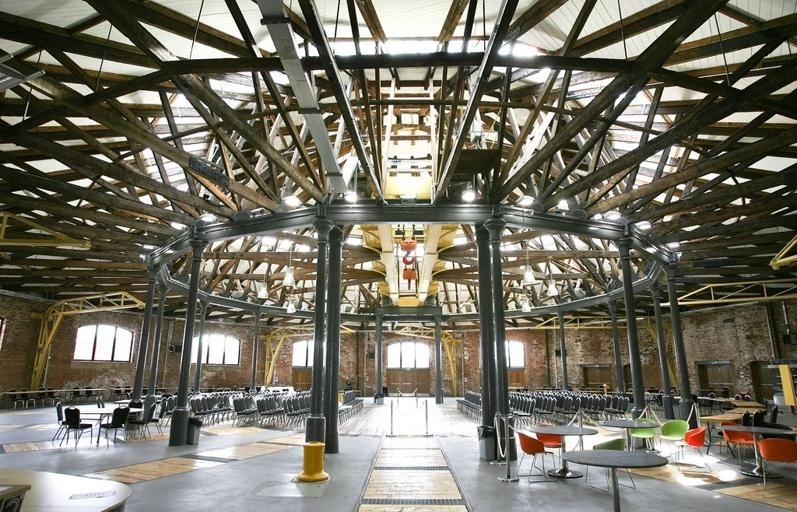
[455,384,796,511]
[1,379,371,450]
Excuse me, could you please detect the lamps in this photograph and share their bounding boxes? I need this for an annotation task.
[521,240,560,316]
[258,241,300,315]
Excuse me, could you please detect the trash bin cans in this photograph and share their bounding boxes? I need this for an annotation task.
[478,425,497,461]
[187,417,202,445]
[375,393,384,405]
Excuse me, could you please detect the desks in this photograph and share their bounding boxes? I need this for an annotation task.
[1,465,134,511]
